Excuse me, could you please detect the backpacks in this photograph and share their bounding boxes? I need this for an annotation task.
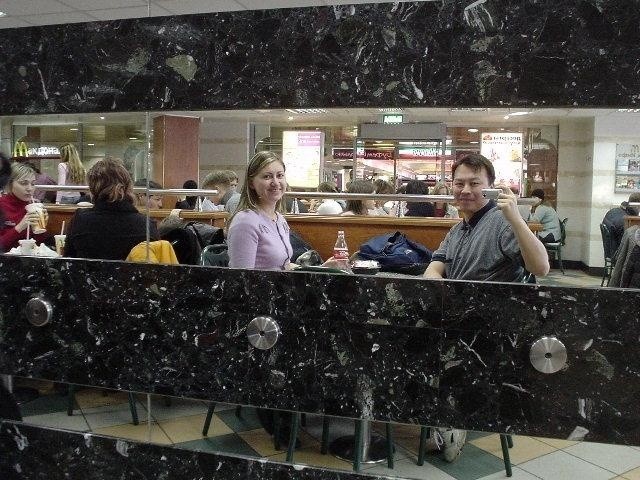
[358,231,433,275]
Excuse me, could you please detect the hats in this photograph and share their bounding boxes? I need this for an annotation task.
[531,189,545,199]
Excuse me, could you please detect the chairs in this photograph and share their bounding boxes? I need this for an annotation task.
[195,243,318,454]
[70,239,181,424]
[540,219,636,290]
[417,262,541,478]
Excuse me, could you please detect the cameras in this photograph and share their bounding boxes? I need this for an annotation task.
[481,188,504,200]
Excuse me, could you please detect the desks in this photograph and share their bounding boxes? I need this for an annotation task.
[302,257,427,465]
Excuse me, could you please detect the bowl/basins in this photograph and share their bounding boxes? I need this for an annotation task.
[350,266,382,276]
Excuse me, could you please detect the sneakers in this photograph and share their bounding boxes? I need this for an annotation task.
[430,425,467,463]
[266,422,301,448]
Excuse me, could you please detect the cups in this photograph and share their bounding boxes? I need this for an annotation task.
[18,239,37,256]
[25,202,48,234]
[54,234,68,256]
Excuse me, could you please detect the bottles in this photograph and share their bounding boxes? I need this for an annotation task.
[331,230,351,266]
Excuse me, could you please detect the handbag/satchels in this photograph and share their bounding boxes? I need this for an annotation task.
[157,226,200,265]
[184,220,225,251]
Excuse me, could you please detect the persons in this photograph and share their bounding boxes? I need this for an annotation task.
[528,188,562,245]
[137,170,242,211]
[282,178,458,218]
[602,192,640,257]
[56,144,88,205]
[228,149,347,449]
[423,154,551,460]
[1,158,157,398]
